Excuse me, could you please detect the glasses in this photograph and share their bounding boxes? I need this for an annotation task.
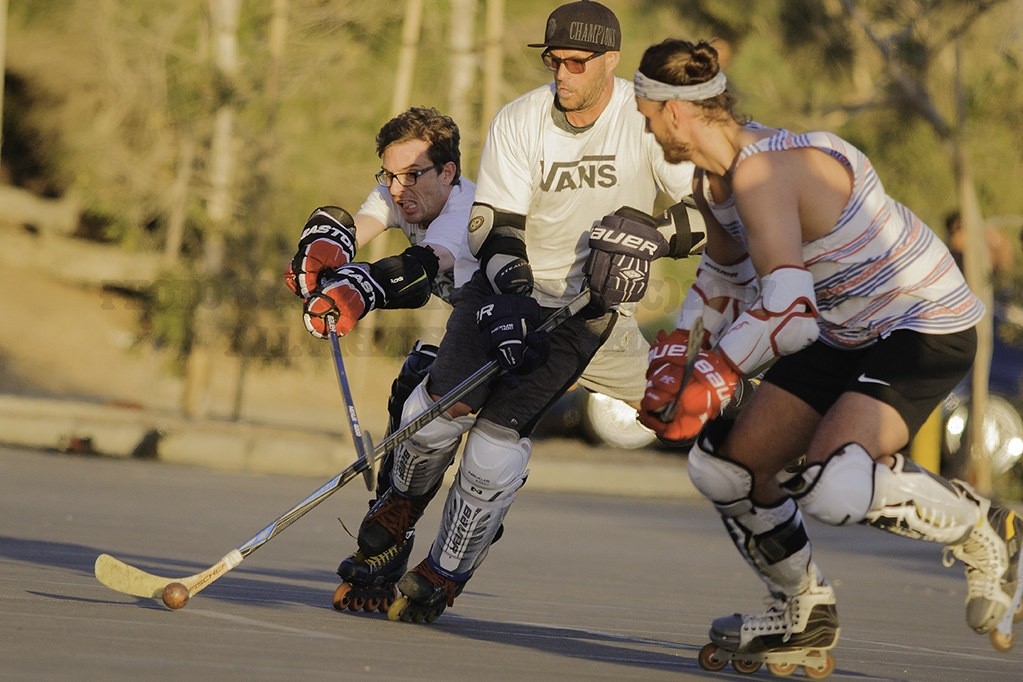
[374,165,436,186]
[542,48,605,74]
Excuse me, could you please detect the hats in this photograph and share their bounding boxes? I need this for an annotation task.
[527,1,621,51]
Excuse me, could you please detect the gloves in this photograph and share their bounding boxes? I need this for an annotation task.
[283,206,358,300]
[465,294,551,376]
[302,263,384,340]
[585,212,670,323]
[634,350,740,442]
[645,329,707,420]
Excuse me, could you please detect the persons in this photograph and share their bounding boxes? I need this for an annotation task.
[633,38,1023,680]
[286,106,1022,651]
[331,0,712,623]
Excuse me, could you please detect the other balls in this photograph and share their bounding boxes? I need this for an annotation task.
[161,582,189,610]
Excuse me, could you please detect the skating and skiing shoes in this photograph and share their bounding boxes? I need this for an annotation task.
[387,527,503,623]
[354,477,446,565]
[945,476,1023,650]
[331,548,408,613]
[698,556,843,678]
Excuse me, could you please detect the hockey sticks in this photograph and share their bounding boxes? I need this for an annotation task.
[324,314,377,496]
[95,288,594,601]
[650,314,706,423]
